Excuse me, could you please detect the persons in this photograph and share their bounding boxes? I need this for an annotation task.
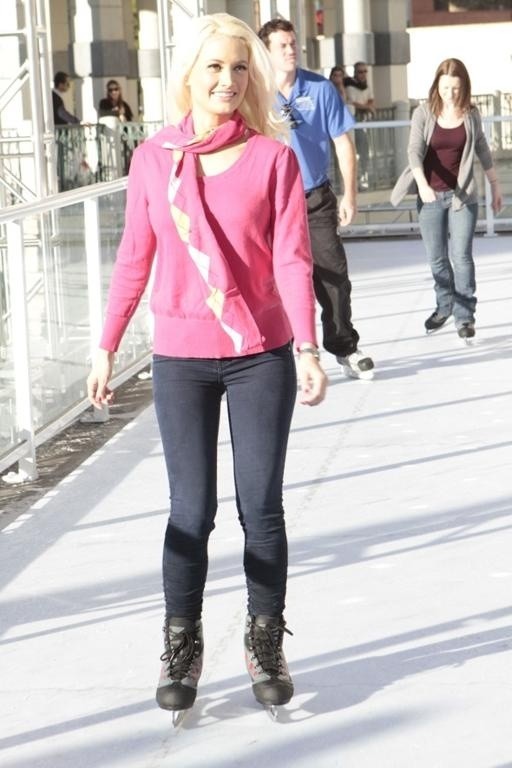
[51,71,142,193]
[85,13,331,709]
[257,20,375,370]
[329,62,378,196]
[389,57,503,340]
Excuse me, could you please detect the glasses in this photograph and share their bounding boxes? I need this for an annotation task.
[108,88,118,92]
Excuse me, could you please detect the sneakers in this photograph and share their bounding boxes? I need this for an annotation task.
[457,319,475,338]
[335,352,375,371]
[424,313,447,329]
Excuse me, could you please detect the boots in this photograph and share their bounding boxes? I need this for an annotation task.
[244,614,293,705]
[157,615,204,711]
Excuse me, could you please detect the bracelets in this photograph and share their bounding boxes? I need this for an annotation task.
[297,347,321,359]
[488,179,499,183]
